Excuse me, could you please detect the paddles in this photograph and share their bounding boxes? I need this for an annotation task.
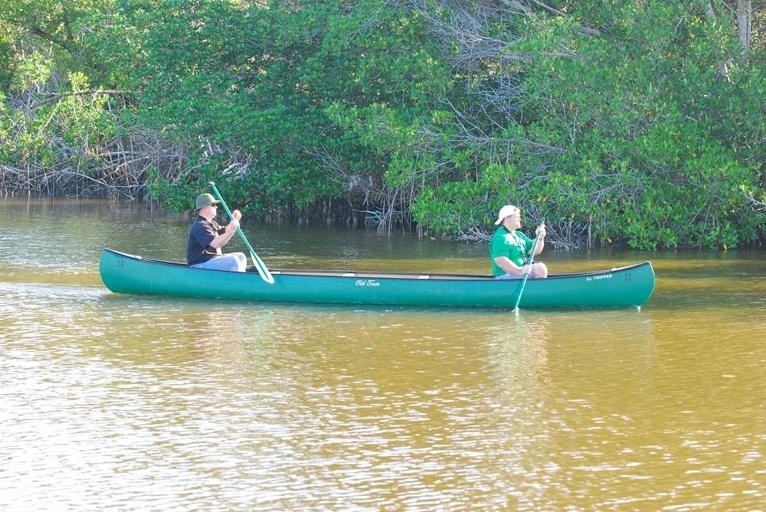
[210,182,274,285]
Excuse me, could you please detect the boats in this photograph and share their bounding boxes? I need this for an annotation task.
[99,247,656,310]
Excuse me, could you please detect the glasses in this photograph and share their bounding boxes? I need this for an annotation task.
[208,203,219,206]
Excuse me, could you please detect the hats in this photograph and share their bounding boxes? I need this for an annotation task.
[494,204,520,226]
[194,193,221,213]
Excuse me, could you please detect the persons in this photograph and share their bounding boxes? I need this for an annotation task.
[488,205,548,279]
[186,192,248,273]
